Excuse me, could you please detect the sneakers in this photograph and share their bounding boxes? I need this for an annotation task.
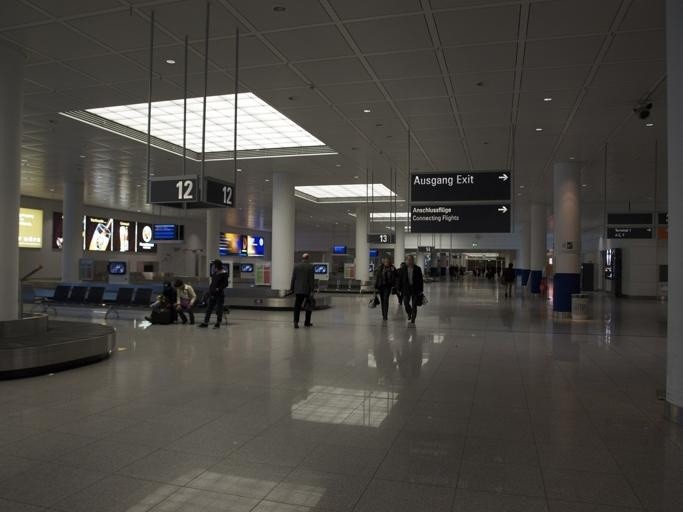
[293,321,313,329]
[407,312,416,323]
[143,315,222,330]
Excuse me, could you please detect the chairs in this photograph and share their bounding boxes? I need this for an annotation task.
[31,284,230,328]
[311,277,377,296]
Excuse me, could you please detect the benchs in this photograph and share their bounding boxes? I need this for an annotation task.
[222,285,332,312]
[0,312,115,376]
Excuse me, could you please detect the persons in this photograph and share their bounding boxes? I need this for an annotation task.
[144,259,228,329]
[373,253,517,324]
[291,253,315,328]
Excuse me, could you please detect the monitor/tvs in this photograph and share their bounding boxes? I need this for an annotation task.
[108,262,126,274]
[241,264,253,272]
[313,264,325,274]
[211,264,229,277]
[370,250,377,257]
[333,245,346,254]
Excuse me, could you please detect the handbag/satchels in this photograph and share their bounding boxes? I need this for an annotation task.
[374,295,381,306]
[391,287,397,295]
[414,293,425,307]
[302,297,317,312]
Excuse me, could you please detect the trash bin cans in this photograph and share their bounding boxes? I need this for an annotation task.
[571,293,590,320]
